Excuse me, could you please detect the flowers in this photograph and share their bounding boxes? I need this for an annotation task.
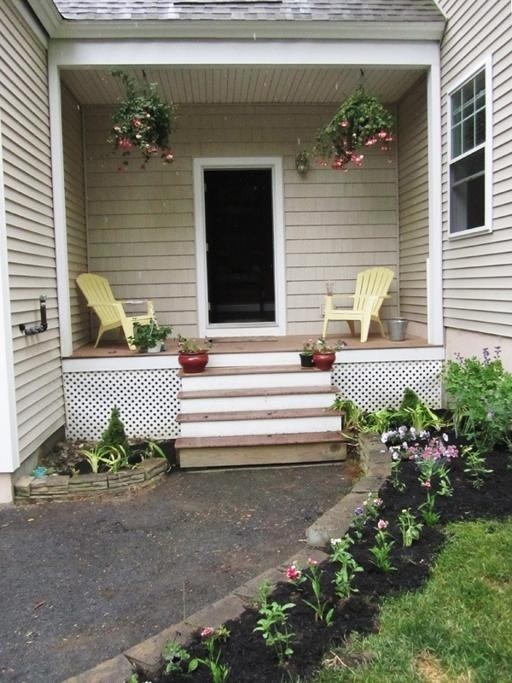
[98,69,176,173]
[313,68,397,172]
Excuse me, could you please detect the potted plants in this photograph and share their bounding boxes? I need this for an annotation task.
[127,318,173,353]
[299,338,314,367]
[177,332,215,372]
[310,338,348,369]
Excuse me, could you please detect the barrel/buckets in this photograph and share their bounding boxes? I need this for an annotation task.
[388,319,408,341]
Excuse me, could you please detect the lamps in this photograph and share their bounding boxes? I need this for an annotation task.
[294,147,310,174]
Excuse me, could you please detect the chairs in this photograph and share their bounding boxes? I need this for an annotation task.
[322,268,396,342]
[74,271,156,350]
